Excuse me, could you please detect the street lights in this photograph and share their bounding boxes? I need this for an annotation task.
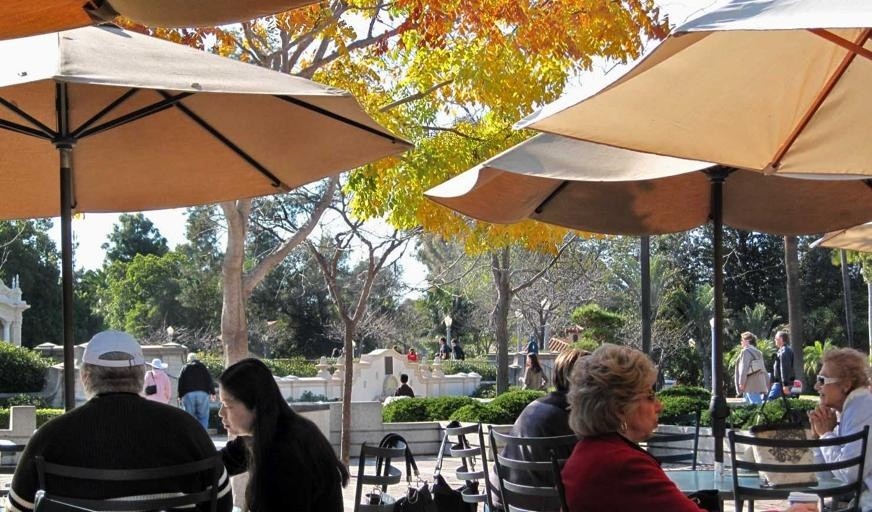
[536,295,555,351]
[513,306,524,351]
[441,312,454,349]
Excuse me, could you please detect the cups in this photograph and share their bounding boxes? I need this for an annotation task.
[788,492,819,508]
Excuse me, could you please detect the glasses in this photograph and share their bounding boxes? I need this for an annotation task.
[817,375,856,388]
[631,390,656,401]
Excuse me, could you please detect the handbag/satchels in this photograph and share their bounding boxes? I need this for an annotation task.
[748,422,818,489]
[146,385,156,395]
[365,484,434,512]
[747,359,762,376]
[429,474,479,512]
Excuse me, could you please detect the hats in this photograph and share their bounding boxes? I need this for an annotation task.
[82,331,145,368]
[145,358,168,370]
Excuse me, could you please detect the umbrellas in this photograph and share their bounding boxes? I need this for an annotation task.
[424,133,872,486]
[1,21,418,414]
[807,219,871,254]
[513,0,872,186]
[0,0,331,44]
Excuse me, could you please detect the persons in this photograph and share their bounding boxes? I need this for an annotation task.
[139,359,171,403]
[5,331,234,512]
[330,347,340,358]
[524,335,538,354]
[517,352,548,392]
[406,347,417,361]
[434,337,451,360]
[393,345,403,354]
[215,358,350,512]
[450,338,465,361]
[764,331,795,401]
[734,331,770,406]
[480,348,591,512]
[804,345,872,511]
[394,374,415,398]
[561,342,818,511]
[176,351,215,432]
